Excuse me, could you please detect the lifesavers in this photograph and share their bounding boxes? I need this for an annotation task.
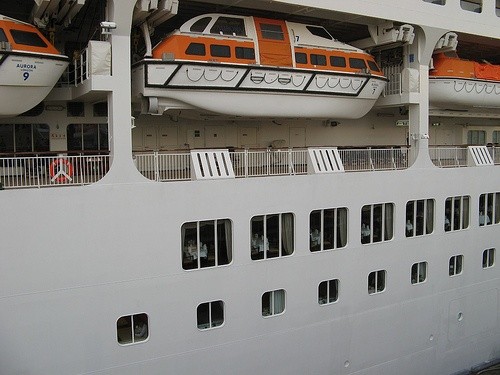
[50,158,73,182]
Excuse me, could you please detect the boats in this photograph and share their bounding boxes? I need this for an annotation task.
[130,14,390,121]
[427,39,500,120]
[0,14,71,117]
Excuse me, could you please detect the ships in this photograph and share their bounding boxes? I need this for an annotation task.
[1,1,500,375]
